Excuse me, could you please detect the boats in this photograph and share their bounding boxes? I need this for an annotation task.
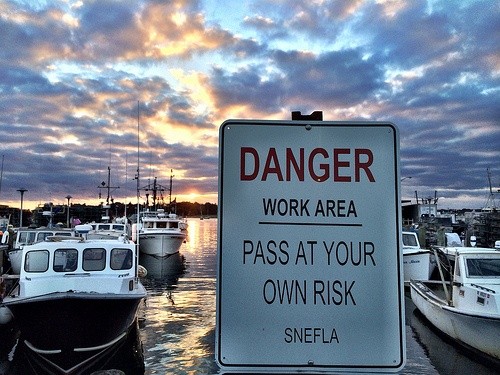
[76,167,132,242]
[0,215,13,232]
[409,240,500,360]
[8,203,79,276]
[403,230,432,287]
[0,230,146,375]
[135,167,189,257]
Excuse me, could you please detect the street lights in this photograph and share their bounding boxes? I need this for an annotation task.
[16,187,29,227]
[65,194,72,229]
